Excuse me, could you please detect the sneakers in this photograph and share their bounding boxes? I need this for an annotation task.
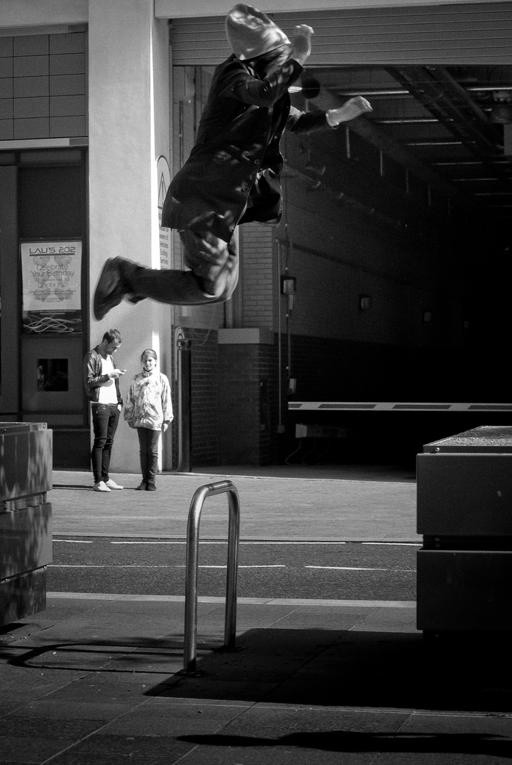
[93,478,124,492]
[135,482,156,491]
[94,256,146,321]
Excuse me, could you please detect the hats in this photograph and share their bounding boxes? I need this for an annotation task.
[225,3,293,59]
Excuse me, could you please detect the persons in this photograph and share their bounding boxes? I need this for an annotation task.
[92,7,371,321]
[125,348,174,491]
[83,326,125,495]
